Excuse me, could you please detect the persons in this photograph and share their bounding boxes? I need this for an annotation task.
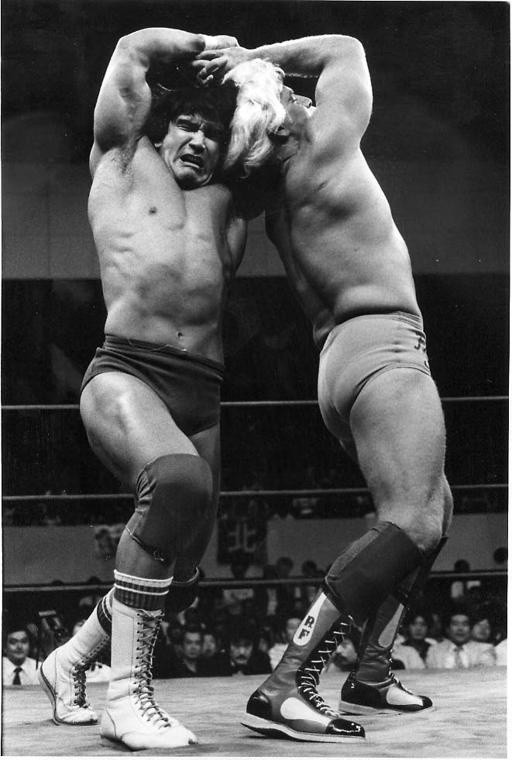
[37,24,267,753]
[190,22,459,749]
[3,553,505,690]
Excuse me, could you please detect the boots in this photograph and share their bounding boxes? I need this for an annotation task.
[339,588,432,717]
[96,597,197,753]
[36,601,108,725]
[240,576,366,744]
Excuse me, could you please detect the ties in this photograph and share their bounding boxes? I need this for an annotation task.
[452,646,463,668]
[13,669,21,685]
